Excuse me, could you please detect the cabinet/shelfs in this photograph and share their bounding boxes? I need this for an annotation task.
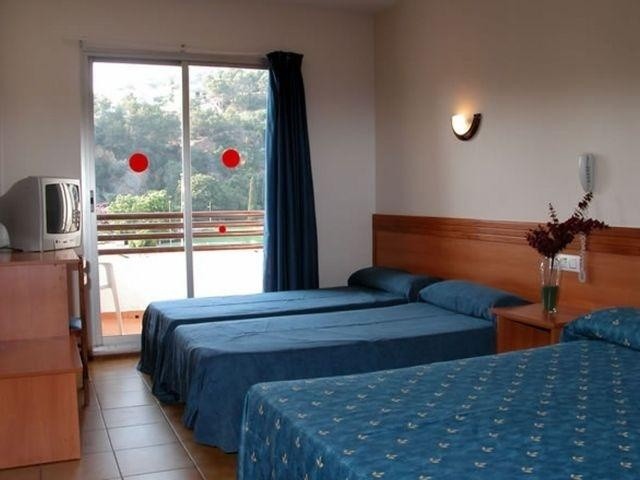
[1,247,88,473]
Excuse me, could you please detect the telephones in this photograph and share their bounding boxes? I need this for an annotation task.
[579,153,595,193]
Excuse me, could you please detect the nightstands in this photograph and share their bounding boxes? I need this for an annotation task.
[489,301,577,356]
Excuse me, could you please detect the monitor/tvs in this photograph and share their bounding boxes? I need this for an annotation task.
[0,177,81,253]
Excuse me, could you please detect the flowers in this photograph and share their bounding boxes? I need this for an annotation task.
[524,191,610,288]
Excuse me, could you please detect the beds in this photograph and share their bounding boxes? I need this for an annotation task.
[250,306,638,478]
[171,279,525,437]
[141,267,443,381]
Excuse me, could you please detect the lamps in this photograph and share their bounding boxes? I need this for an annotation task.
[449,113,482,141]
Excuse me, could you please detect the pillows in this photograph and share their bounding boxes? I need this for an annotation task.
[348,267,438,301]
[418,280,528,321]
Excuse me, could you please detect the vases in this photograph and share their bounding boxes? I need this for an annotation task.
[538,255,565,311]
